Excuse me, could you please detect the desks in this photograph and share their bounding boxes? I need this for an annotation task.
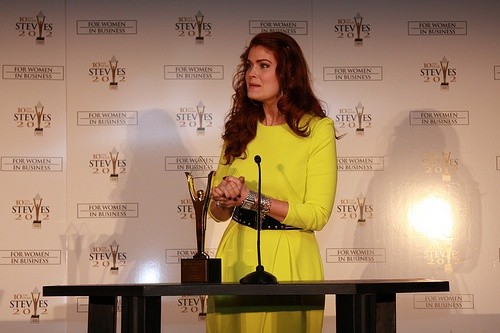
[43,278,450,333]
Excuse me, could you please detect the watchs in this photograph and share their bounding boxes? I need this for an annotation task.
[240,190,255,210]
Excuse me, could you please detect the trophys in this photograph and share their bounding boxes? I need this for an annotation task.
[181,171,221,284]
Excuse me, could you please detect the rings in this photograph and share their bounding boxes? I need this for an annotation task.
[215,201,221,207]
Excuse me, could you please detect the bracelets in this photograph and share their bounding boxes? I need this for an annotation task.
[251,193,272,219]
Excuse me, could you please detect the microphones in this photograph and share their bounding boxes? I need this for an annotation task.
[239,155,278,285]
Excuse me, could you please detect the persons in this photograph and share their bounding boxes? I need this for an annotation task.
[205,33,338,333]
